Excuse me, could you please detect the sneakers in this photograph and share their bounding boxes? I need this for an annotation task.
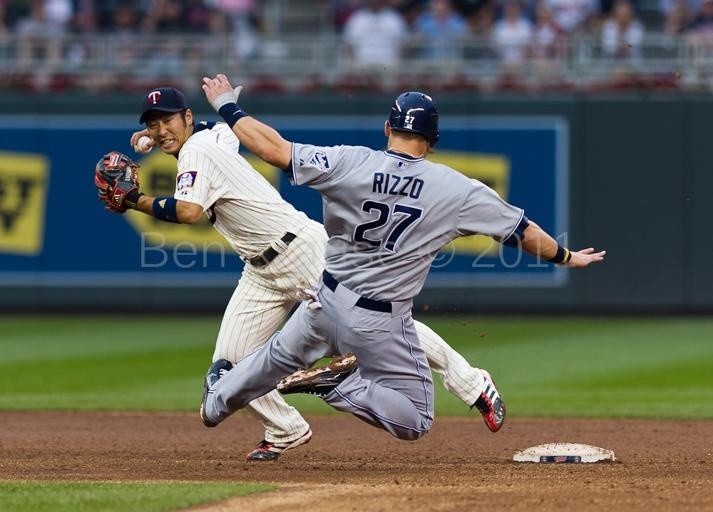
[200,359,233,428]
[275,352,358,398]
[246,429,313,461]
[474,369,504,433]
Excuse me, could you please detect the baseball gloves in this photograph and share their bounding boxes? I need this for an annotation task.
[94,151,139,214]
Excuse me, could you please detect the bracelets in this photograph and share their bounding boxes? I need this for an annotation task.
[153,195,178,223]
[547,243,572,266]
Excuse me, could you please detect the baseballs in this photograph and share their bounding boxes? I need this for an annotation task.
[134,136,152,154]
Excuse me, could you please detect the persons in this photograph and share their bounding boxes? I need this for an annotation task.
[93,86,507,462]
[199,72,607,441]
[0,0,262,94]
[336,1,713,90]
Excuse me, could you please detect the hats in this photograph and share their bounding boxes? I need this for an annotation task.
[139,86,187,125]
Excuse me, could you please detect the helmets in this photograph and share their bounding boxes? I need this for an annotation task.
[389,91,440,147]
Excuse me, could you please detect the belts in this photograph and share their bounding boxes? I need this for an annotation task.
[322,270,392,313]
[244,232,296,266]
